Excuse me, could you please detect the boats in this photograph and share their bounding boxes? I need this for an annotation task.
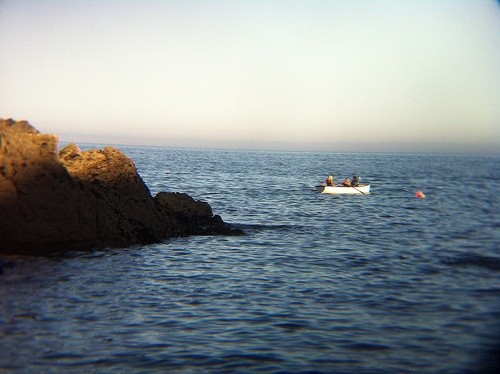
[315,183,369,195]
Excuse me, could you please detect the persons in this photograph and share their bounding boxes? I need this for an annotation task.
[327,175,335,187]
[342,177,351,187]
[351,175,359,187]
